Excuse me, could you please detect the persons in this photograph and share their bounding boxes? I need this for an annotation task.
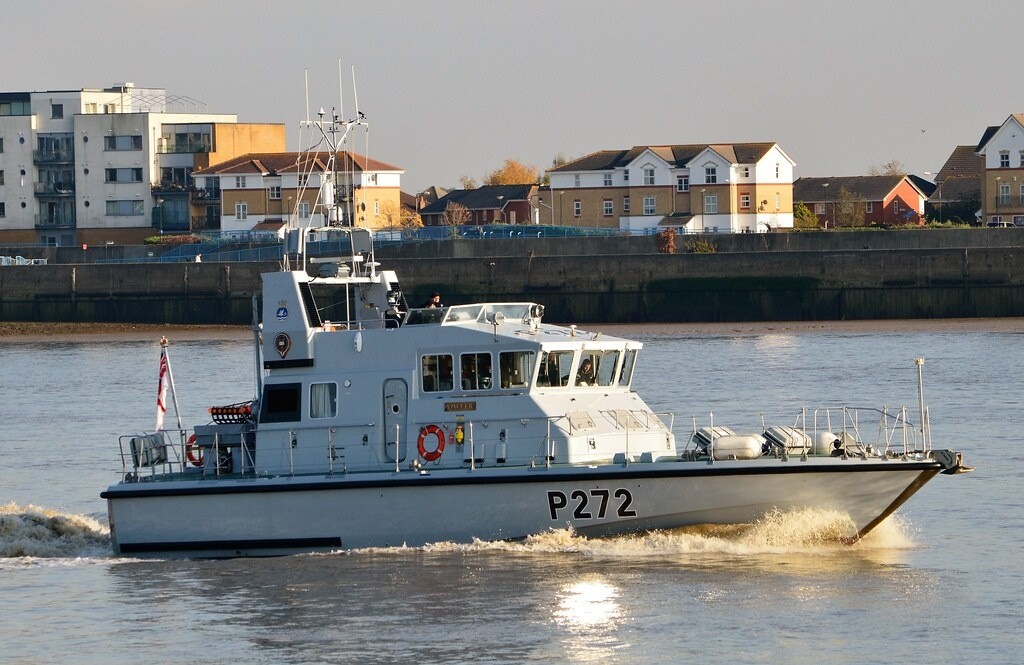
[422,292,444,324]
[576,359,593,384]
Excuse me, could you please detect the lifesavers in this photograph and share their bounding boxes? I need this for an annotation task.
[417,425,445,459]
[185,434,203,465]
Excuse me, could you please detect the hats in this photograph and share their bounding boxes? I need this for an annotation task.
[432,292,440,298]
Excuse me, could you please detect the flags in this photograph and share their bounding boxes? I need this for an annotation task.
[155,349,168,431]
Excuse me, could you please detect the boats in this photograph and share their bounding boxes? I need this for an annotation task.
[100,105,971,560]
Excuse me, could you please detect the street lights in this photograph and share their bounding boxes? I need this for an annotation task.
[995,177,1000,227]
[822,183,829,227]
[157,199,164,243]
[559,191,565,225]
[699,188,706,230]
[725,179,733,232]
[496,196,504,223]
[924,172,942,224]
[288,196,292,232]
[415,191,430,212]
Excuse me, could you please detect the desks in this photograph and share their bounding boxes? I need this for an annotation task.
[194,424,252,474]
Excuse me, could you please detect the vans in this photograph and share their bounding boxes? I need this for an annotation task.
[987,222,1017,227]
